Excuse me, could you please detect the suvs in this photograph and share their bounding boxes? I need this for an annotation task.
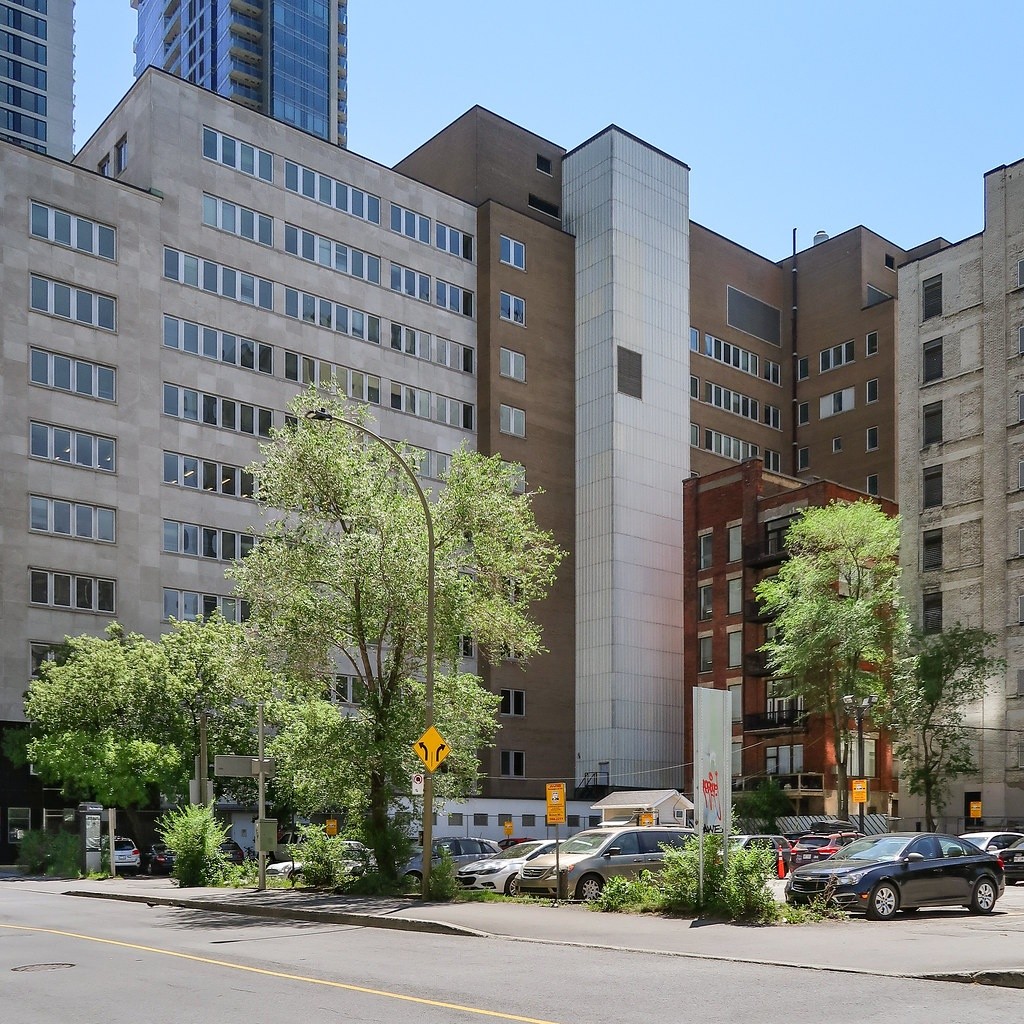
[789,830,866,873]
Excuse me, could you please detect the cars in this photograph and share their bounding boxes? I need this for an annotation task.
[265,841,369,883]
[108,836,141,877]
[396,832,503,889]
[455,840,567,898]
[141,843,177,875]
[717,835,792,878]
[218,837,244,865]
[942,832,1024,857]
[498,835,538,850]
[999,836,1024,885]
[784,832,1005,921]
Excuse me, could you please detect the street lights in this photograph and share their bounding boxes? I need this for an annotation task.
[306,407,434,899]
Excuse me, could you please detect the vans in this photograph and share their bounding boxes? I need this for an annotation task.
[515,827,699,905]
[266,831,329,865]
